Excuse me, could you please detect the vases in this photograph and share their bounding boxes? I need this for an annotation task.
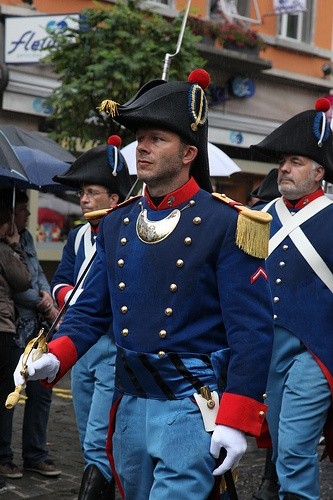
[223,38,261,56]
[195,33,214,45]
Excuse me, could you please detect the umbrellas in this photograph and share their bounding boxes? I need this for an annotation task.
[120,140,241,178]
[0,125,78,188]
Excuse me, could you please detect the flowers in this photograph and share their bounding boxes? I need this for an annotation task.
[179,13,267,52]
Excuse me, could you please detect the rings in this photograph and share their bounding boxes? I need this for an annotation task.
[47,303,51,307]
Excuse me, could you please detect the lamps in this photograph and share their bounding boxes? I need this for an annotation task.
[319,63,332,80]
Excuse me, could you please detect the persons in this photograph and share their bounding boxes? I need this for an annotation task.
[250,168,282,211]
[51,136,131,500]
[14,69,272,500]
[0,205,30,489]
[0,186,62,477]
[250,97,333,500]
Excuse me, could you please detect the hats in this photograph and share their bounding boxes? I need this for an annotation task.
[248,98,333,185]
[52,134,135,203]
[96,69,214,193]
[248,168,282,202]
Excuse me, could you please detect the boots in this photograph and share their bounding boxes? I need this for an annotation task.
[78,464,104,500]
[101,475,116,500]
[252,449,288,500]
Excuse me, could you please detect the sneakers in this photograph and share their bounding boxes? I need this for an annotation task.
[0,460,22,478]
[22,459,62,475]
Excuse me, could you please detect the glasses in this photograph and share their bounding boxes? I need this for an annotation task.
[75,188,113,198]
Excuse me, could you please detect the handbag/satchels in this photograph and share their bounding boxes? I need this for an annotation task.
[12,315,54,349]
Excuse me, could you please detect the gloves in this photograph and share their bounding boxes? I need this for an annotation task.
[64,289,84,307]
[13,347,60,395]
[210,424,248,476]
